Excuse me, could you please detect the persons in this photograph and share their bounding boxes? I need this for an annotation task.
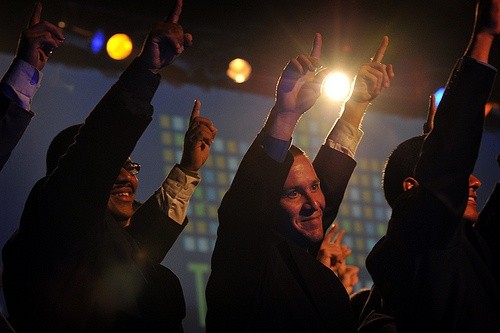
[206,33,357,333]
[357,95,436,332]
[1,0,67,174]
[307,36,396,257]
[365,0,500,333]
[473,153,500,263]
[119,100,216,264]
[0,0,193,333]
[318,223,360,297]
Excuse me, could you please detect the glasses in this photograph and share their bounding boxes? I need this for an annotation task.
[123,163,140,175]
